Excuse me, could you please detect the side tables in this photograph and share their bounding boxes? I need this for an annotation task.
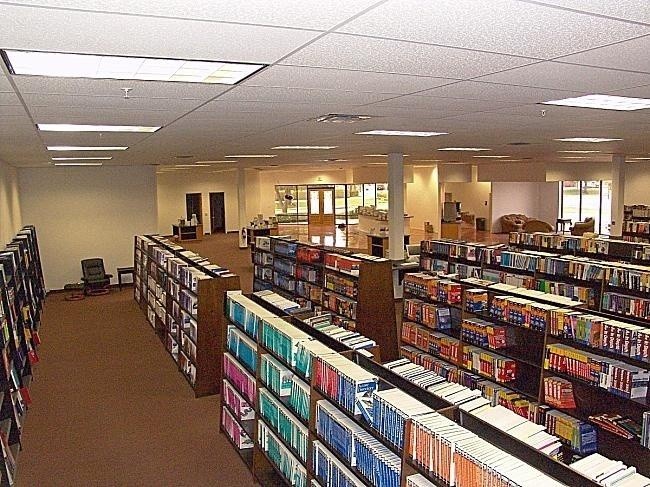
[117,267,135,293]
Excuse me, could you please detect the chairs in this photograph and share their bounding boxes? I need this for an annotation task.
[82,257,112,296]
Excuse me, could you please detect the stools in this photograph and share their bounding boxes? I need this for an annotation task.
[64,279,89,301]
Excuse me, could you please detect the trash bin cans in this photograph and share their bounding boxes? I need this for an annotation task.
[424,221,432,232]
[476,217,486,231]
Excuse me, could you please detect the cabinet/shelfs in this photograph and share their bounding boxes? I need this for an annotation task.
[620,206,649,240]
[216,289,649,485]
[418,234,648,324]
[133,231,243,399]
[509,226,650,266]
[0,223,47,487]
[391,266,649,472]
[247,233,403,365]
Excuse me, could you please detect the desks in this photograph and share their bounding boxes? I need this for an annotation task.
[170,214,206,242]
[237,213,281,245]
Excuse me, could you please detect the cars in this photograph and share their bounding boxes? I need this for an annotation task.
[587,181,600,189]
[377,184,386,190]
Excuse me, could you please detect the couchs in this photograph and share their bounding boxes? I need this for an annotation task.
[499,210,599,234]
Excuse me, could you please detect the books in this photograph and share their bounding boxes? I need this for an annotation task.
[221,233,649,487]
[135,234,234,386]
[362,205,389,219]
[0,224,47,487]
[622,205,650,242]
